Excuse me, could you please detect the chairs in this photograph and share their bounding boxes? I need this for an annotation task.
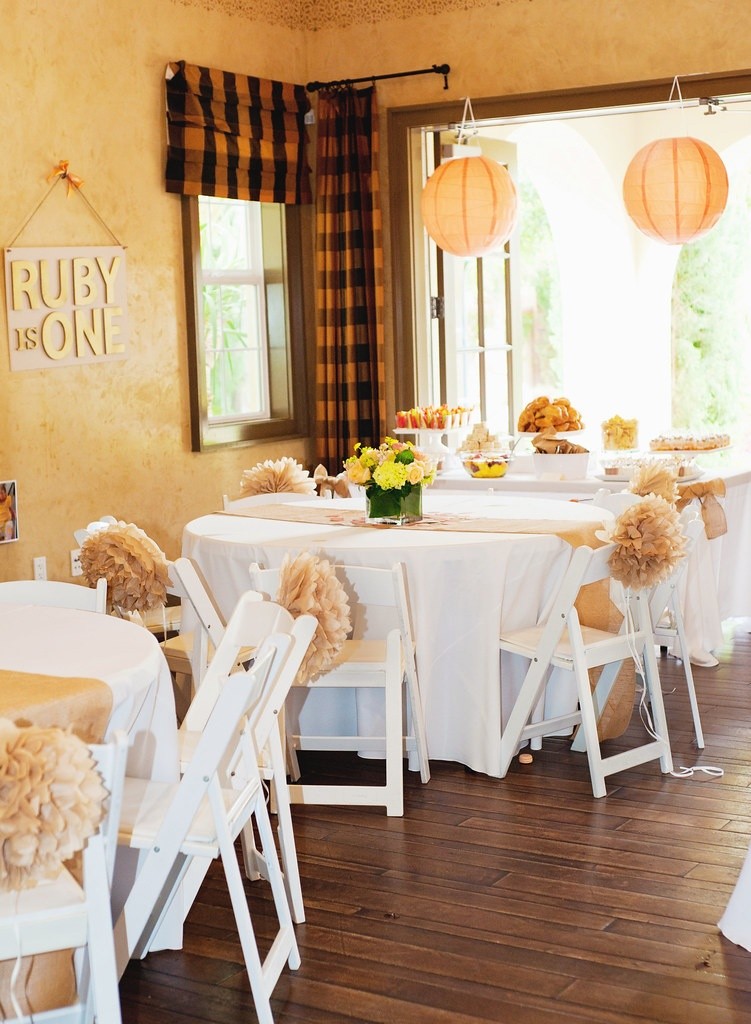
[160,587,318,924]
[496,535,676,804]
[218,486,333,512]
[85,527,306,783]
[638,504,714,749]
[75,519,194,634]
[99,630,305,1024]
[0,727,139,1024]
[334,468,366,500]
[247,556,434,819]
[0,577,107,614]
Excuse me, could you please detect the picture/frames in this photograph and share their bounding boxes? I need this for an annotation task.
[0,480,20,544]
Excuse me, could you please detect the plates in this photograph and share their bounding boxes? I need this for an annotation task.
[649,443,733,456]
[595,470,704,483]
[518,428,586,438]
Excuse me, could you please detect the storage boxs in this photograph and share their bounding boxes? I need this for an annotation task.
[534,450,591,479]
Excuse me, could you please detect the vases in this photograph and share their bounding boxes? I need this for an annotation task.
[363,477,425,524]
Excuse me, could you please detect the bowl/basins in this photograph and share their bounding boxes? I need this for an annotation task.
[533,452,589,477]
[457,451,517,478]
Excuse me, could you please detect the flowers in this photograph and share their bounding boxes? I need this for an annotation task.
[0,721,112,892]
[270,551,355,685]
[341,436,439,492]
[239,454,317,498]
[608,492,684,591]
[627,464,686,503]
[76,527,175,614]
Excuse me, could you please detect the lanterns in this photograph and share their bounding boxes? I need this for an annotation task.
[623,137,728,244]
[421,156,517,257]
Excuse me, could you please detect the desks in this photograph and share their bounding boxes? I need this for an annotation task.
[0,600,173,1005]
[396,468,751,662]
[178,492,639,780]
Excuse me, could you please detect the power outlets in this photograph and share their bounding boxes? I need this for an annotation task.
[70,548,83,576]
[32,556,47,581]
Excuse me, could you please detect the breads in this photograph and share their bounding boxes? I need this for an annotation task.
[458,421,501,450]
[518,396,585,433]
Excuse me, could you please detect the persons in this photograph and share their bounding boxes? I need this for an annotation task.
[0,483,16,541]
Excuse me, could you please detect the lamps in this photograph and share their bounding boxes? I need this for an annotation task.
[416,88,525,260]
[623,76,734,247]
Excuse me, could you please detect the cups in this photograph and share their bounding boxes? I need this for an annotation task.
[393,427,469,459]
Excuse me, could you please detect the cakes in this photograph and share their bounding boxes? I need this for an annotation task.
[648,433,730,450]
[603,455,697,478]
